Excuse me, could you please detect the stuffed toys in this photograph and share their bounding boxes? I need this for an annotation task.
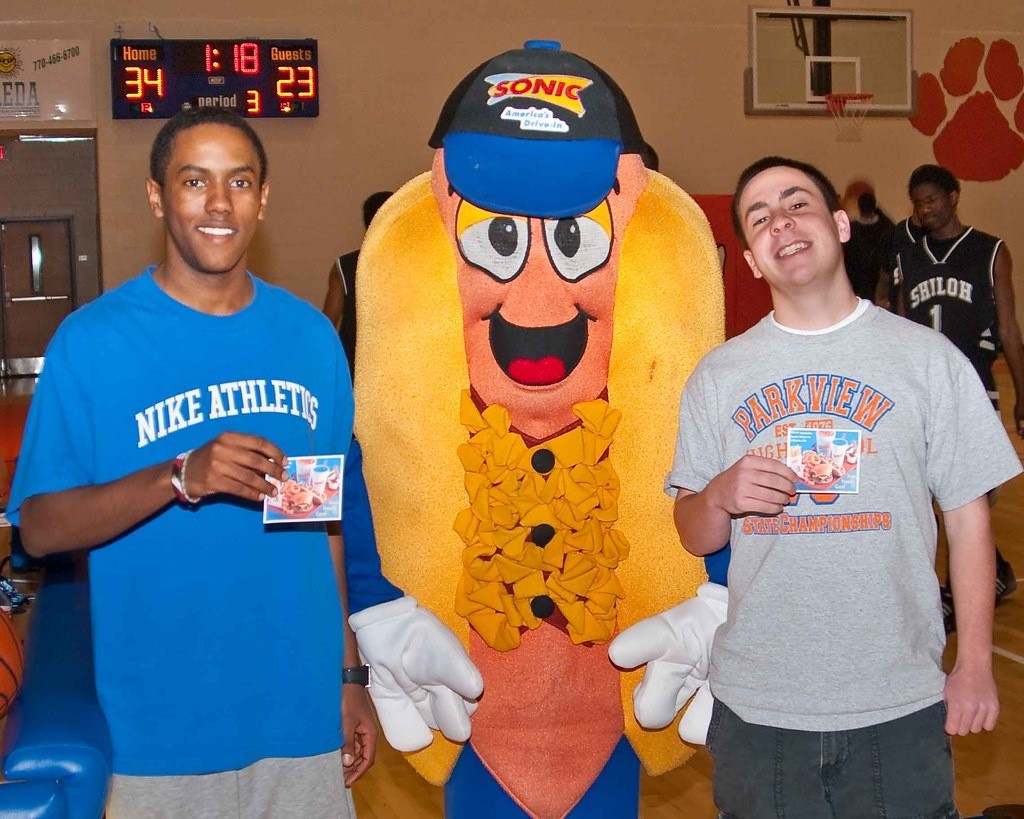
[339,40,731,819]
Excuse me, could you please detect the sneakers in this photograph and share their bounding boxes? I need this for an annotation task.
[940,585,956,635]
[995,560,1016,604]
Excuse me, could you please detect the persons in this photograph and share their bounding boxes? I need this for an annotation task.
[844,192,896,303]
[664,157,1024,819]
[897,164,1023,636]
[887,202,922,318]
[322,191,396,384]
[6,110,378,819]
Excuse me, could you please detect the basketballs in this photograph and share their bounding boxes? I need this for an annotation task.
[1,606,24,720]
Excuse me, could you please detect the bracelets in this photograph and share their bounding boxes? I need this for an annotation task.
[171,449,200,503]
[343,663,372,688]
